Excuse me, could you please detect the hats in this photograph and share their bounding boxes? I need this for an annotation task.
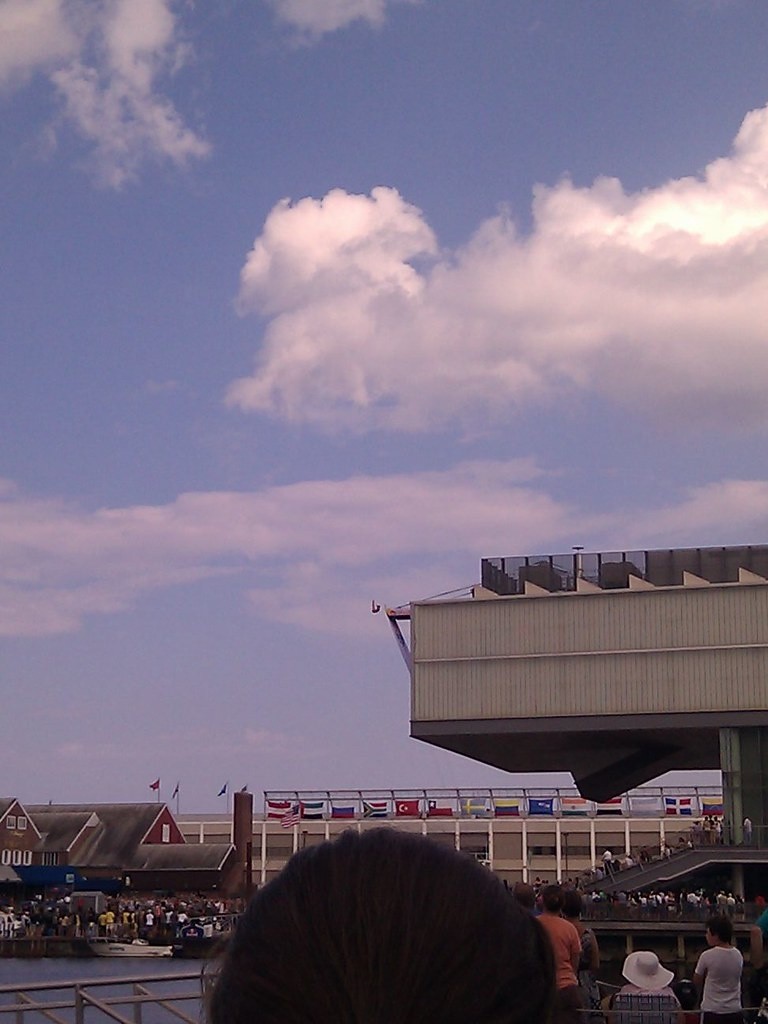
[623,950,674,988]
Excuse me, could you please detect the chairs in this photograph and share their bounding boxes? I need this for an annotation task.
[609,992,682,1024]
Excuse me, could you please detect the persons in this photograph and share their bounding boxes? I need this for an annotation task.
[532,884,582,992]
[607,949,686,1024]
[750,905,768,1024]
[197,828,556,1024]
[0,884,250,954]
[508,817,767,918]
[556,890,605,1022]
[690,914,747,1024]
[546,980,596,1024]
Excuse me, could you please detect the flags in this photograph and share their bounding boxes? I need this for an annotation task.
[458,797,491,817]
[493,796,521,817]
[662,795,694,817]
[279,804,300,828]
[529,795,555,817]
[630,796,662,818]
[239,785,247,794]
[394,800,420,817]
[361,798,390,819]
[300,799,325,819]
[425,798,455,817]
[149,779,159,792]
[560,795,588,817]
[595,794,623,817]
[266,797,290,819]
[330,805,356,821]
[699,796,725,816]
[217,783,227,797]
[172,782,178,800]
